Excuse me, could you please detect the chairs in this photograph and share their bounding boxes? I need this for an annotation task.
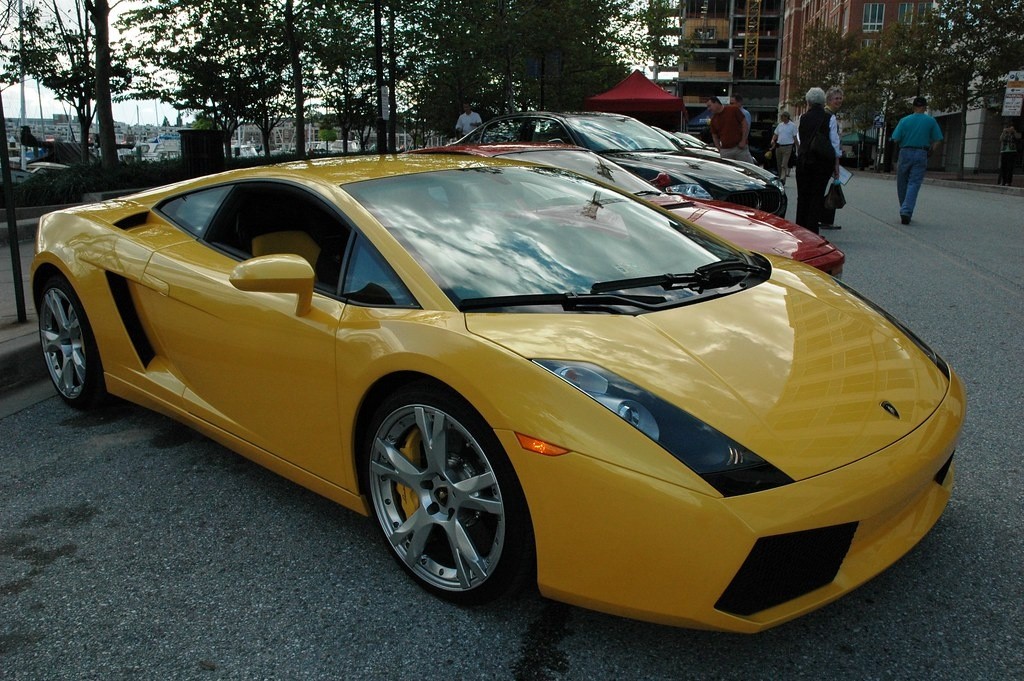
[251,230,321,281]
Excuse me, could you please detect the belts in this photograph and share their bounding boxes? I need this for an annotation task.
[915,146,930,151]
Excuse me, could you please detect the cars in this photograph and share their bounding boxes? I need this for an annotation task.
[650,124,760,167]
[440,110,788,220]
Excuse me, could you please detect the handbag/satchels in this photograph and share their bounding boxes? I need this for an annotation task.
[827,180,846,209]
[810,112,835,162]
[764,150,772,160]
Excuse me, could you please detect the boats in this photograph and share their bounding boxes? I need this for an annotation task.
[0,75,361,183]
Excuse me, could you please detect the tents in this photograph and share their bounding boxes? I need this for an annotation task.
[840,131,878,168]
[583,69,689,133]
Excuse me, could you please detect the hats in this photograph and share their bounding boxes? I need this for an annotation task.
[913,97,928,107]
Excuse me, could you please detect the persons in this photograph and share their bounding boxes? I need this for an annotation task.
[891,97,943,224]
[729,95,751,151]
[455,102,482,143]
[704,96,753,164]
[771,111,800,185]
[999,126,1017,186]
[818,86,844,229]
[792,87,842,234]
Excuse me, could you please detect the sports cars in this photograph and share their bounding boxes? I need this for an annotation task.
[28,152,968,639]
[403,141,846,284]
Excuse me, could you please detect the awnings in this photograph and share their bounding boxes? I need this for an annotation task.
[687,107,767,128]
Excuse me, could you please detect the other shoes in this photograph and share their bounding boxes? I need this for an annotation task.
[900,213,911,225]
[821,223,841,230]
